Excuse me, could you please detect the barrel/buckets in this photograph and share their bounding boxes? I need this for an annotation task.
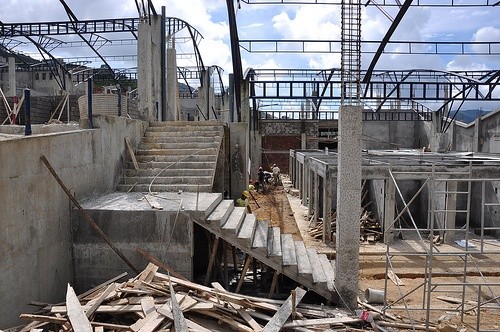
[364,288,385,304]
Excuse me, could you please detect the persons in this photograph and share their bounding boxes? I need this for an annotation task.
[272,164,280,186]
[258,167,265,191]
